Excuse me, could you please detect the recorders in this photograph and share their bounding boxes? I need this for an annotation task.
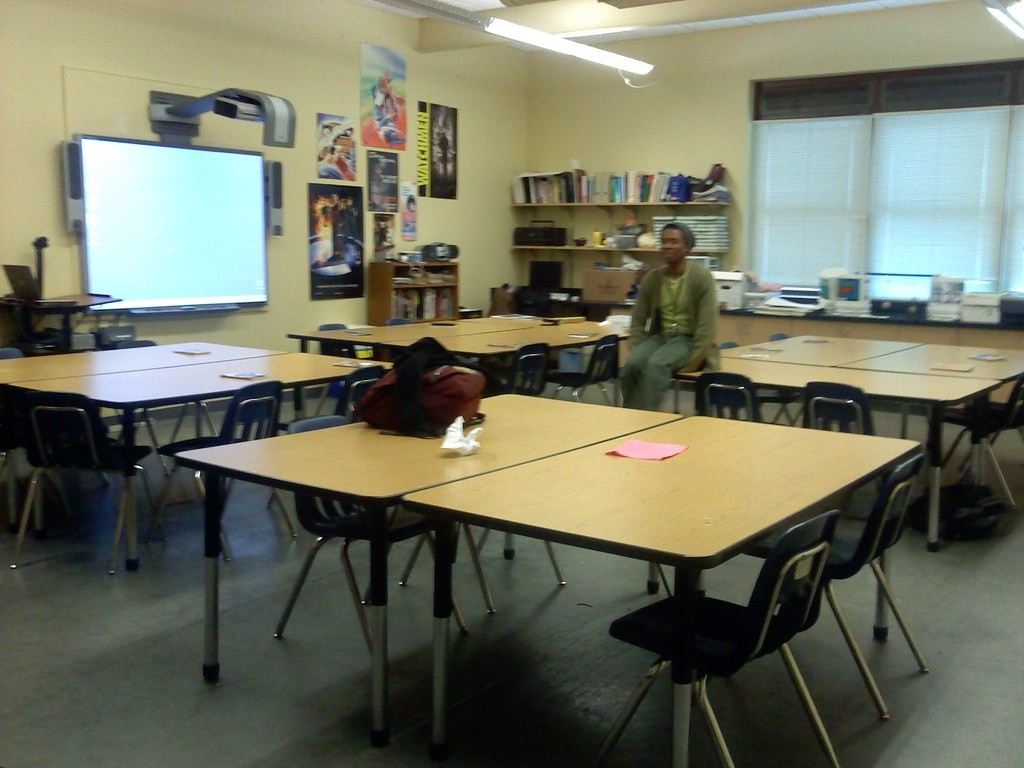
[415,242,459,262]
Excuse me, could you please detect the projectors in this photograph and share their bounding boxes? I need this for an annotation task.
[213,96,264,122]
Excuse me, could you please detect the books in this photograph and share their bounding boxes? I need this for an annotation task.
[391,288,454,320]
[513,160,731,203]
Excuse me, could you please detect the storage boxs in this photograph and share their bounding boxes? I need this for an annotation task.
[584,269,637,300]
[710,271,753,310]
[960,292,1024,326]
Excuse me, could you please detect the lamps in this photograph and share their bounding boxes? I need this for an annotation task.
[371,0,655,77]
[982,0,1024,38]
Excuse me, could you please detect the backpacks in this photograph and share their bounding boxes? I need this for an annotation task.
[354,338,487,433]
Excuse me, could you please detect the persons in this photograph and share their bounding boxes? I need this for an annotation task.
[623,223,724,410]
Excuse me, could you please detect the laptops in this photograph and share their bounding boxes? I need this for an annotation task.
[2,265,78,303]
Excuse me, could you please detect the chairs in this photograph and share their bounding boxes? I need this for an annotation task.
[0,318,1024,768]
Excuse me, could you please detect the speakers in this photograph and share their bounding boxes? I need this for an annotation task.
[267,160,284,236]
[63,141,84,232]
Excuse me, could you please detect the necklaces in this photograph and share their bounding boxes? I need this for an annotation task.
[665,275,683,337]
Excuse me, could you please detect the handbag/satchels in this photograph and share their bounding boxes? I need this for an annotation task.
[907,481,1010,543]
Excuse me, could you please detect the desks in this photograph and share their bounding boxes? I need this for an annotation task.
[401,415,922,768]
[288,314,632,376]
[0,341,290,383]
[173,395,684,748]
[0,293,123,357]
[839,344,1024,485]
[0,352,394,571]
[719,335,924,367]
[668,357,1002,552]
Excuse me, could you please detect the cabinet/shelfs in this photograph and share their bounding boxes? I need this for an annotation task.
[369,261,460,361]
[510,200,732,253]
[610,306,1024,416]
